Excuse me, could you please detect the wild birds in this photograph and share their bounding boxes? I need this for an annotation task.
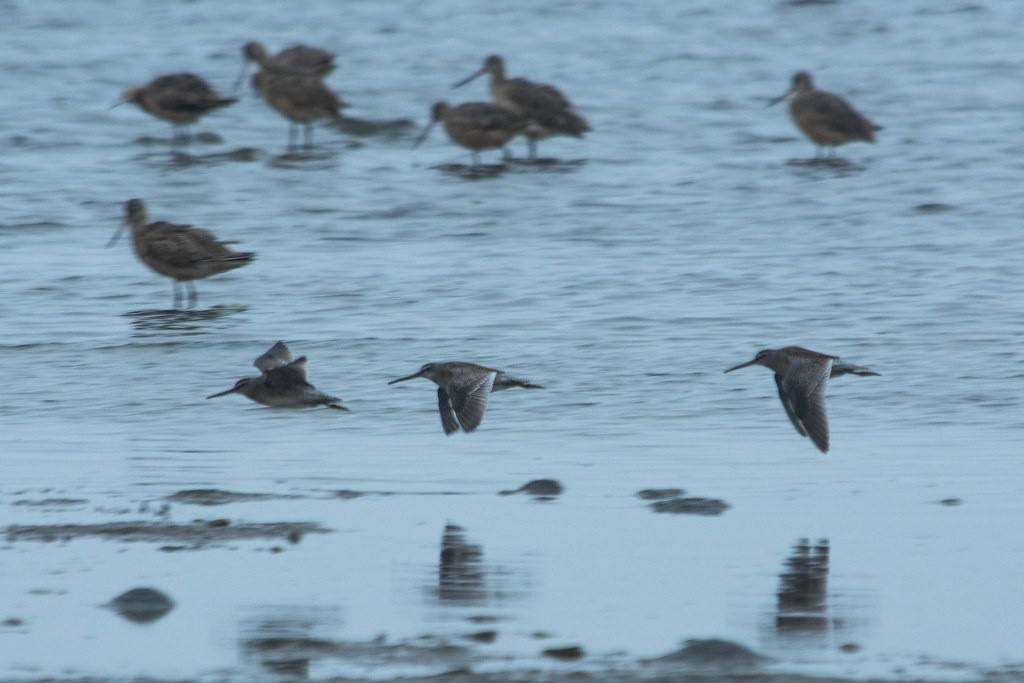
[767,71,884,159]
[206,340,351,413]
[453,53,592,160]
[387,360,546,435]
[104,197,260,311]
[724,344,881,454]
[109,73,240,144]
[242,42,354,153]
[414,98,538,171]
